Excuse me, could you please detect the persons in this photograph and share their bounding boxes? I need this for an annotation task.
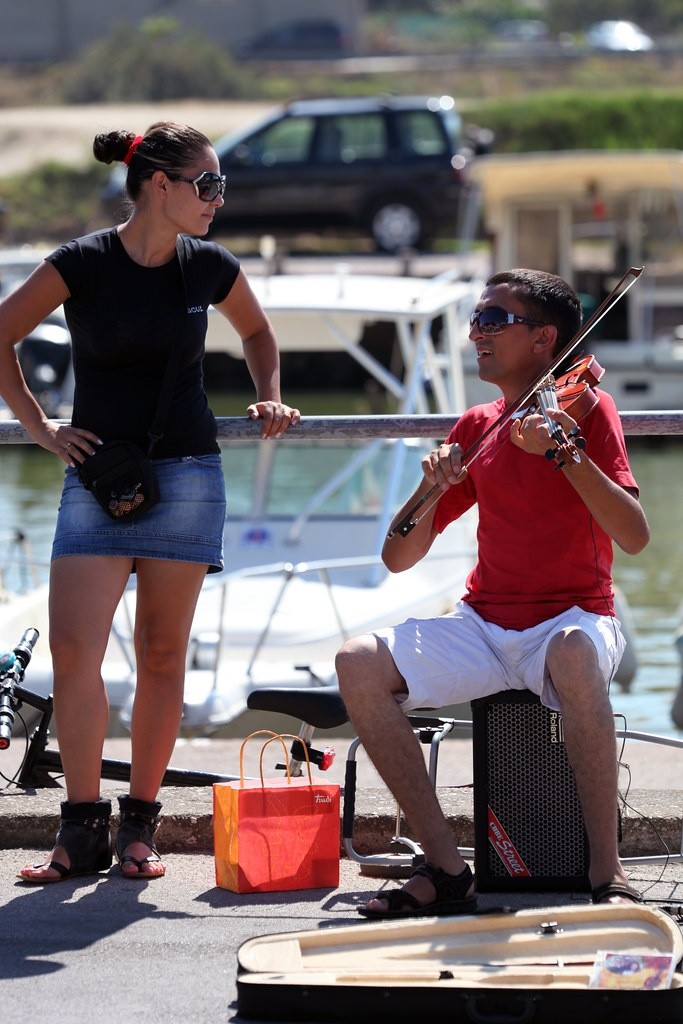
[0,121,300,885]
[336,266,648,920]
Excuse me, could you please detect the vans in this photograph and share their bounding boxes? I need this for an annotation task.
[102,95,482,248]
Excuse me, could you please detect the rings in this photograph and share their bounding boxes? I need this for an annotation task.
[64,441,71,449]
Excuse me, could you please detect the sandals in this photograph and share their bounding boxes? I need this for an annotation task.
[591,883,643,906]
[356,862,477,916]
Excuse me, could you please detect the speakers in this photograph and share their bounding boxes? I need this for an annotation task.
[471,688,607,892]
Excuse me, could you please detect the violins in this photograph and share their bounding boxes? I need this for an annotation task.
[502,356,608,477]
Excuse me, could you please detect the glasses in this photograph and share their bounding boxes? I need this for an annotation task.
[468,306,544,335]
[163,171,227,203]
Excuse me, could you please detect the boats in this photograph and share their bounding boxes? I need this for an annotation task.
[0,273,486,740]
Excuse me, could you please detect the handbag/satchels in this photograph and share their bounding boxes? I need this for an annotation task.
[212,728,341,894]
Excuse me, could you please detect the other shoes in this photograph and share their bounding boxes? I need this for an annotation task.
[115,793,166,878]
[16,798,114,884]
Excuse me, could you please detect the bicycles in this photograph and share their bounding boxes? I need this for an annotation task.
[0,629,350,788]
